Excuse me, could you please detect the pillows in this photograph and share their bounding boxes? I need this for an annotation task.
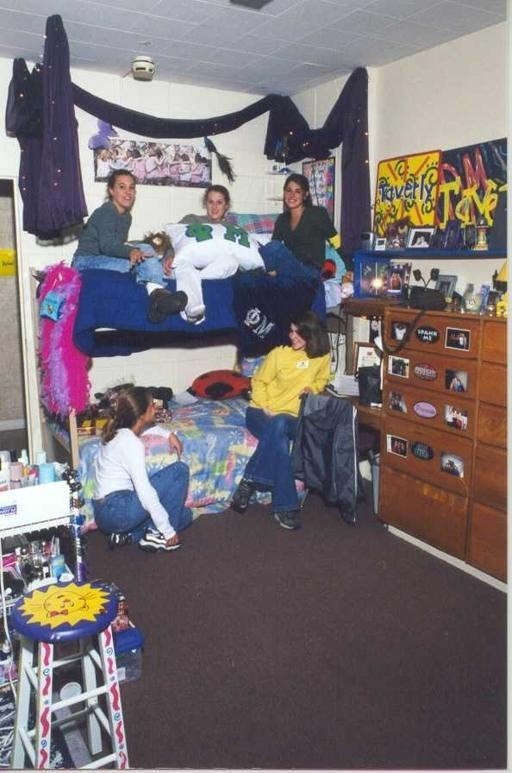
[226,214,282,234]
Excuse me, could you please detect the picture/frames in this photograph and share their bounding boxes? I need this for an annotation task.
[384,261,412,299]
[406,225,438,249]
[434,273,457,298]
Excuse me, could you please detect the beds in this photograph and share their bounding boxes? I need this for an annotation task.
[33,265,349,537]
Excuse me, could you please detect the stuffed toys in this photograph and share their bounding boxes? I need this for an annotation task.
[142,229,175,275]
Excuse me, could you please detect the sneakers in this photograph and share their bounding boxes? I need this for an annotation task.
[138,527,183,552]
[145,287,189,324]
[274,511,296,530]
[107,531,144,547]
[232,479,252,513]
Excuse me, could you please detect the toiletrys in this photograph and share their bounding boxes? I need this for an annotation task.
[0,444,57,492]
[0,535,68,599]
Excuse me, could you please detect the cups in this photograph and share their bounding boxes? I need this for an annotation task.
[464,292,484,312]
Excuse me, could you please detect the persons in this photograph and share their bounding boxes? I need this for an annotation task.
[447,374,464,391]
[370,315,377,340]
[453,411,463,430]
[392,322,407,340]
[392,361,405,374]
[390,440,406,455]
[451,407,458,428]
[419,235,429,247]
[411,236,419,246]
[258,171,347,292]
[454,332,467,349]
[91,383,197,554]
[228,308,334,529]
[389,396,404,411]
[174,185,237,228]
[441,458,459,475]
[390,270,402,290]
[71,169,188,326]
[99,145,210,184]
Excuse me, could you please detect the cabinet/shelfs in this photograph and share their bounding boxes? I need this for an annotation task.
[341,297,506,585]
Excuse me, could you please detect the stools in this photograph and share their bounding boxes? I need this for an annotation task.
[12,583,130,770]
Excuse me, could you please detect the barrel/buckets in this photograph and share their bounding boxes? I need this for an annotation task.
[368,452,380,514]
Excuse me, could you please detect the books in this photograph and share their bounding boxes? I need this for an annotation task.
[327,374,360,397]
[323,385,349,400]
